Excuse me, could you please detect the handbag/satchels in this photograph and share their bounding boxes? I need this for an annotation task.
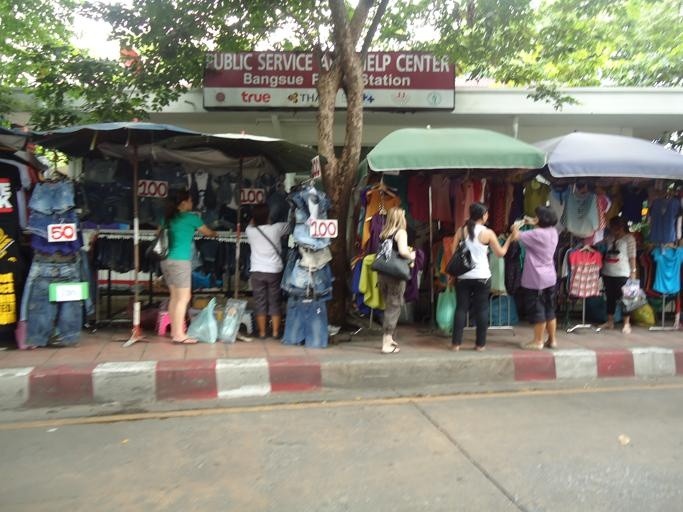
[144,217,168,262]
[370,227,411,281]
[620,288,647,314]
[444,226,476,277]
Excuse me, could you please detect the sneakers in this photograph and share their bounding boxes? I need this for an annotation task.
[621,323,631,334]
[519,340,543,350]
[597,320,613,330]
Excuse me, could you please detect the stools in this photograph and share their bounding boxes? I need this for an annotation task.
[240,309,256,335]
[154,312,187,337]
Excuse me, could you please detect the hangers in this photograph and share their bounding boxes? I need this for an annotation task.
[561,240,595,254]
[293,288,320,302]
[83,228,251,244]
[363,202,388,222]
[38,254,75,264]
[646,241,677,250]
[415,170,521,188]
[546,178,682,200]
[366,176,397,198]
[498,232,509,241]
[38,268,70,282]
[48,161,68,179]
[295,179,324,201]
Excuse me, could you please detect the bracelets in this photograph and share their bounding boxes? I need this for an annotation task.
[630,267,637,274]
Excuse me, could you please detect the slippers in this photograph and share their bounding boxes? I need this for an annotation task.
[171,338,199,344]
[381,345,399,353]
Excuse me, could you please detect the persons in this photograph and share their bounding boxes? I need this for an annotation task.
[446,201,520,352]
[244,203,294,339]
[155,190,220,345]
[598,215,638,334]
[376,204,416,354]
[510,206,560,351]
[121,41,145,78]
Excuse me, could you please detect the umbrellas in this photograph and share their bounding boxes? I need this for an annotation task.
[522,127,679,329]
[24,117,210,341]
[146,130,326,340]
[353,123,545,335]
